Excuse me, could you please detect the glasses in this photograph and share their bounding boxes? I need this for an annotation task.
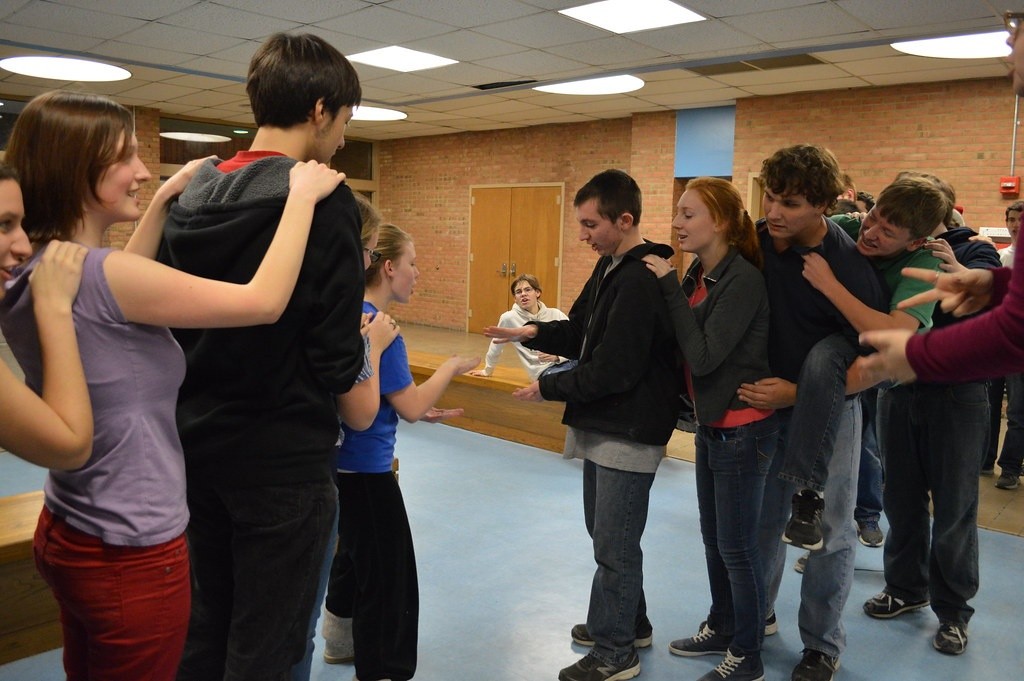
[365,246,382,261]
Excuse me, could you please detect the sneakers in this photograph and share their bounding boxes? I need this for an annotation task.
[995,471,1020,490]
[571,617,652,648]
[558,653,641,681]
[697,645,765,681]
[781,490,824,551]
[669,622,729,655]
[934,620,968,653]
[765,615,776,636]
[863,592,930,618]
[981,464,996,474]
[795,555,808,571]
[854,508,883,547]
[791,649,839,680]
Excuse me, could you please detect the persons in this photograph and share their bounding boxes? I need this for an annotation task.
[699,145,1024,681]
[289,188,400,681]
[321,224,481,681]
[154,33,364,681]
[483,168,682,681]
[468,273,578,385]
[0,149,93,470]
[643,177,778,681]
[0,89,346,681]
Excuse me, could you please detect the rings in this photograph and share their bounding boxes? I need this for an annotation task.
[389,320,397,330]
[934,271,940,285]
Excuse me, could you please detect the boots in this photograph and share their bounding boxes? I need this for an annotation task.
[324,608,356,663]
